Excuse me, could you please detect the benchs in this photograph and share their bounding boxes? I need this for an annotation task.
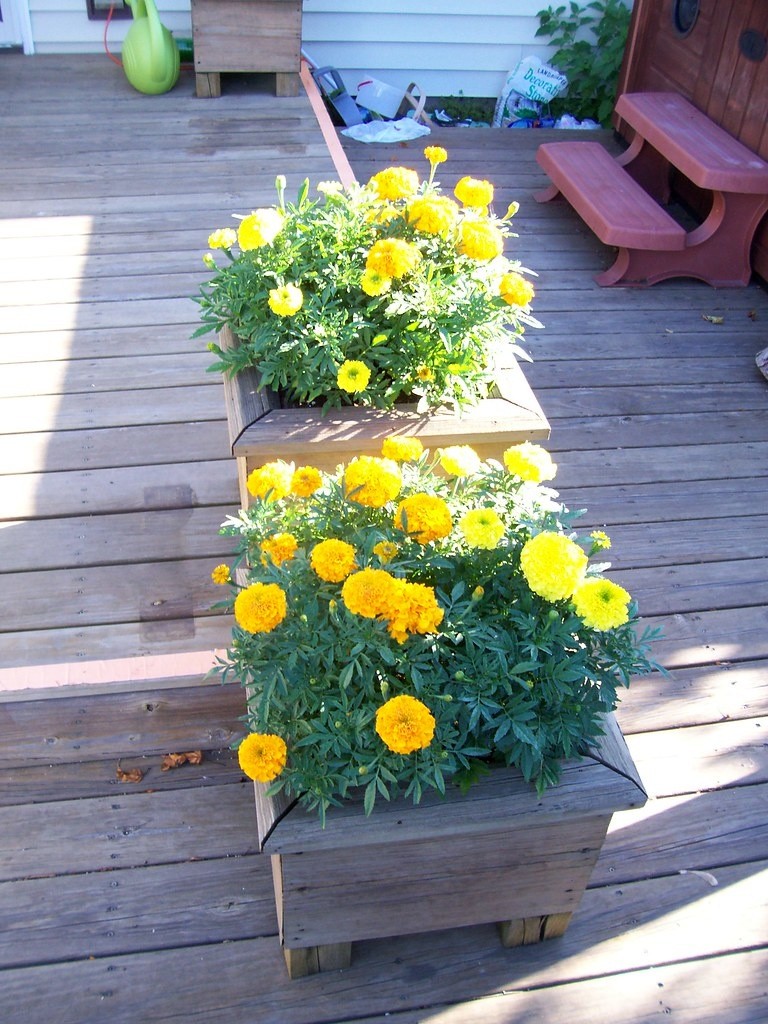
[532,92,768,292]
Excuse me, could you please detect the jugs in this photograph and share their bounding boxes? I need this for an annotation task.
[119,0,181,95]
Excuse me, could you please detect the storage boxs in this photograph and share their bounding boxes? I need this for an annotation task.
[356,76,412,121]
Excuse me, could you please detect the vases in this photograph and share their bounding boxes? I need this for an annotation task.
[214,306,551,562]
[190,0,304,100]
[238,569,646,980]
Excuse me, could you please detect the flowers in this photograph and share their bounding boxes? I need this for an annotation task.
[190,144,546,416]
[200,432,677,831]
